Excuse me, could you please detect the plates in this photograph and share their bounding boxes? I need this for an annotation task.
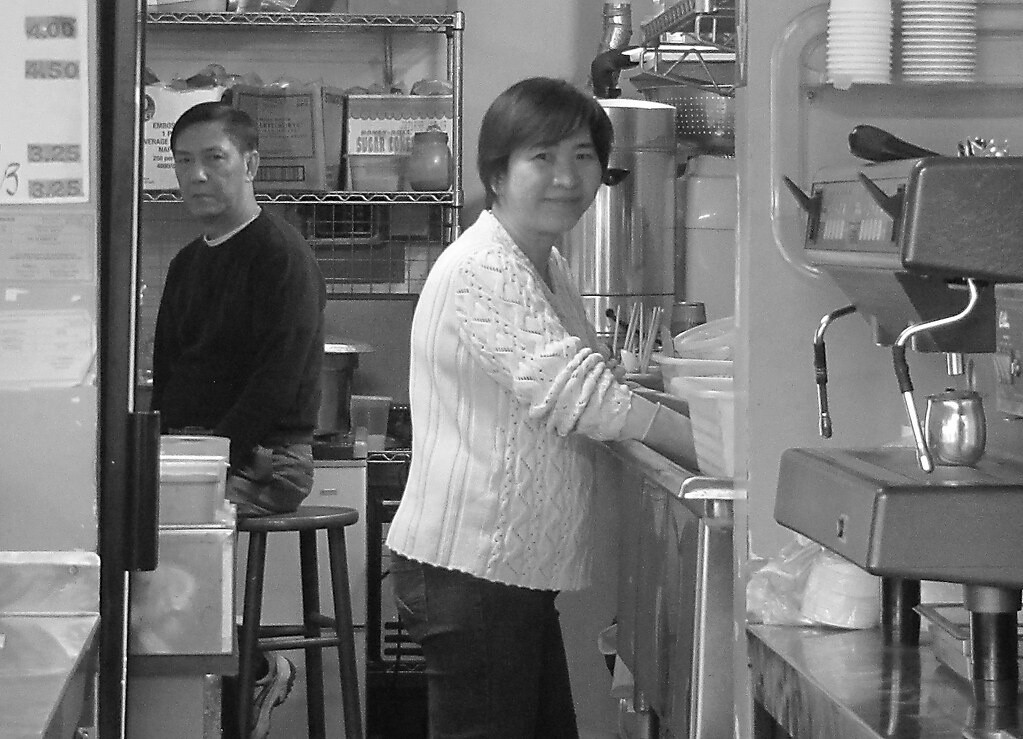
[826,0,895,84]
[901,0,977,82]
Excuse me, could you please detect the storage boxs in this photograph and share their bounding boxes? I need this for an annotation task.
[341,92,453,194]
[138,78,231,193]
[228,85,350,193]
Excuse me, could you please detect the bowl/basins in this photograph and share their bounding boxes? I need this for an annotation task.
[800,554,881,630]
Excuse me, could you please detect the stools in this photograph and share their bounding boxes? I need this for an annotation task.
[220,507,365,739]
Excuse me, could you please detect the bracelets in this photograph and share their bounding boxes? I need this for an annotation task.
[641,402,661,441]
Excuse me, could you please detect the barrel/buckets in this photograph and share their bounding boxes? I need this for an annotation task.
[675,317,734,361]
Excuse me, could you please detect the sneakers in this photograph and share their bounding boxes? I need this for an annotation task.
[245,654,295,739]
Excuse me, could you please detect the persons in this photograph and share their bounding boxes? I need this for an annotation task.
[384,77,700,739]
[149,102,329,739]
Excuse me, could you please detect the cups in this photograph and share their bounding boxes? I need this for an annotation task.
[351,395,393,453]
[671,301,707,341]
[923,387,987,466]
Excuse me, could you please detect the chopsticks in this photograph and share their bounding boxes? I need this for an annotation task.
[612,302,664,373]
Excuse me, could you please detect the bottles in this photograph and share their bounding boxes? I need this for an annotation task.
[409,131,451,195]
